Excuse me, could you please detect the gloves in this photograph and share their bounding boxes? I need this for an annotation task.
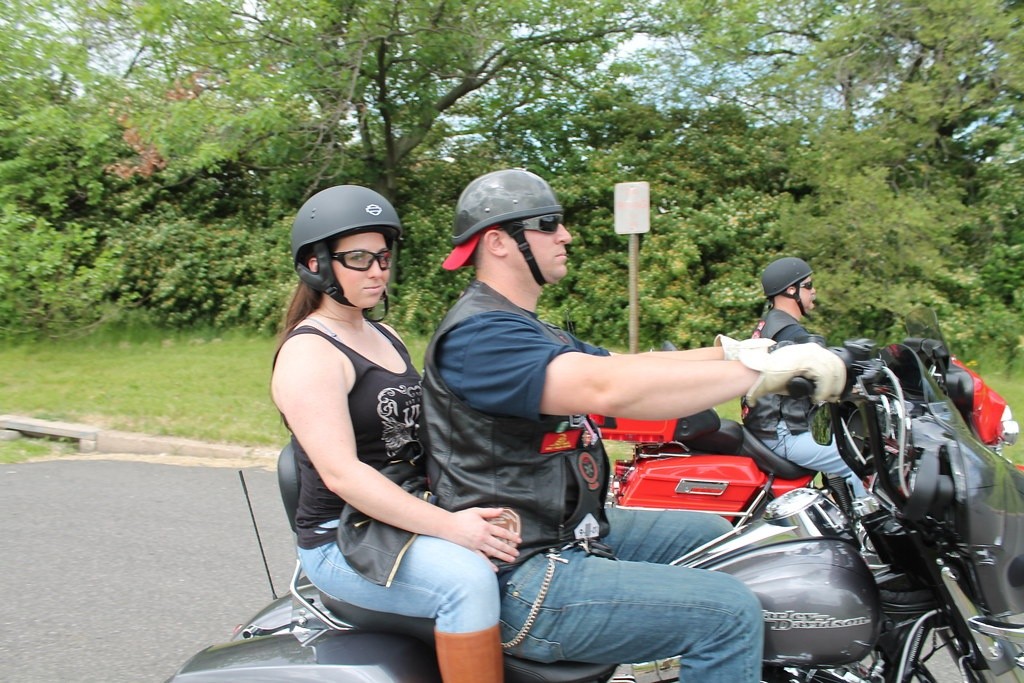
[714,334,778,361]
[736,342,846,408]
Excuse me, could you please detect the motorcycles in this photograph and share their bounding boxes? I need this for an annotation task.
[163,305,1024,683]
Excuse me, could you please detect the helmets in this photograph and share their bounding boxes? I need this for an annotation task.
[451,169,563,246]
[761,257,813,296]
[292,184,403,292]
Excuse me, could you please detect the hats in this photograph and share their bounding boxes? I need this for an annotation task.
[442,223,501,271]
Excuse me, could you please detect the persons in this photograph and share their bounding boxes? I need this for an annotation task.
[271,185,521,683]
[419,170,846,683]
[742,257,868,502]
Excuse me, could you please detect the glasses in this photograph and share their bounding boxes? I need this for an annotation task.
[800,281,812,289]
[499,213,563,233]
[330,250,391,271]
